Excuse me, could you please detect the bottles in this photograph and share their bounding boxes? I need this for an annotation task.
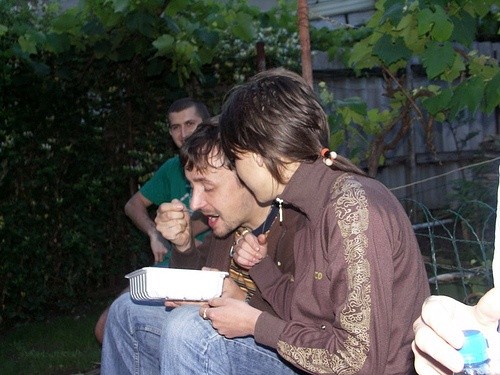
[453,330,493,375]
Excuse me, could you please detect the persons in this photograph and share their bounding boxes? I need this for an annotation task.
[410,253,499,374]
[96,113,311,345]
[100,66,433,374]
[123,97,214,268]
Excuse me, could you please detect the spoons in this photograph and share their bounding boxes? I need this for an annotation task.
[181,210,218,218]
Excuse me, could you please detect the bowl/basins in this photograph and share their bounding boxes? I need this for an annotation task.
[125,267,229,303]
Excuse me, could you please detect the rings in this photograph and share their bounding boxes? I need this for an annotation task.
[235,237,245,245]
[241,229,248,236]
[202,307,208,320]
